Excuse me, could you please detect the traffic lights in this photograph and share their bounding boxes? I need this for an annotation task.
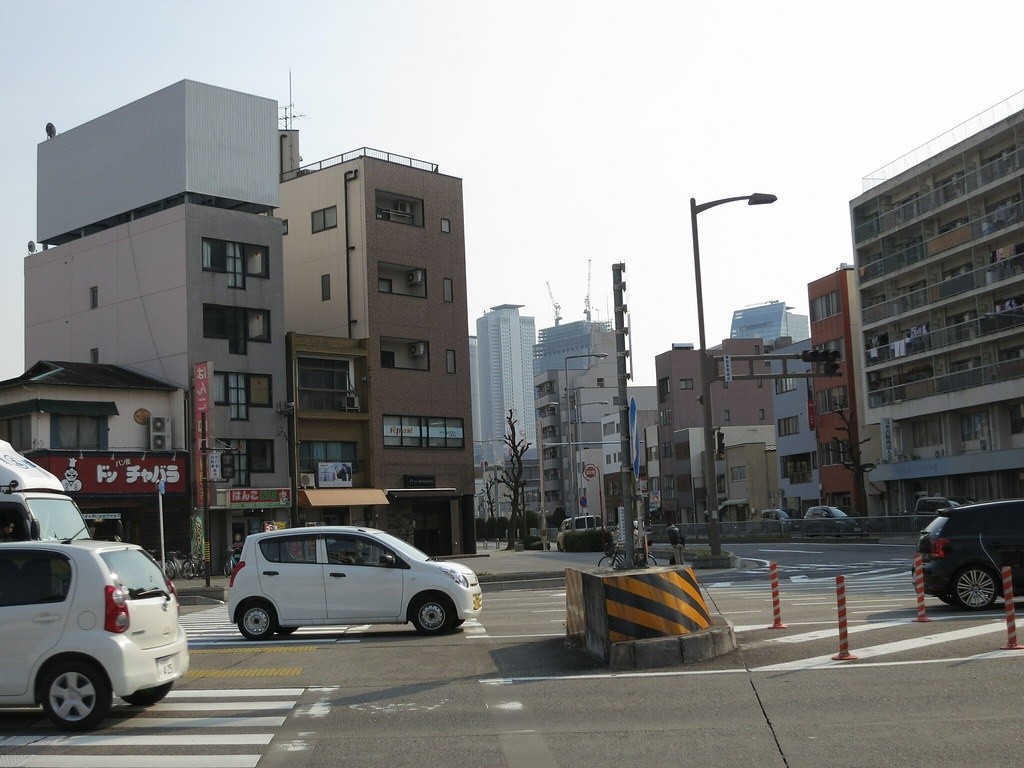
[824,362,840,375]
[717,432,725,460]
[220,453,236,478]
[802,348,841,363]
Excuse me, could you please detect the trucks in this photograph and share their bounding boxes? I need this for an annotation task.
[0,440,94,543]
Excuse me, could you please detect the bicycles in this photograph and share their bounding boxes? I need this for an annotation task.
[149,549,208,581]
[223,546,240,578]
[597,539,657,568]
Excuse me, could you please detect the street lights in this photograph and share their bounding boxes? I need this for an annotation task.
[564,353,608,533]
[691,193,778,556]
[549,400,608,517]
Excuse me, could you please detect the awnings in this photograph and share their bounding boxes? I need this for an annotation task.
[298,489,390,507]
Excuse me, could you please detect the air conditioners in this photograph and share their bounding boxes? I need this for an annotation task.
[405,270,421,285]
[979,437,987,450]
[900,454,915,462]
[408,342,424,358]
[340,396,359,408]
[932,449,944,458]
[299,473,315,487]
[394,200,410,214]
[146,416,172,451]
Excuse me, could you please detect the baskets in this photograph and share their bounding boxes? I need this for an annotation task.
[604,542,615,556]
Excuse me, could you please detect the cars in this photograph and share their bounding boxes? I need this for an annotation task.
[0,540,190,732]
[610,520,653,546]
[557,514,606,552]
[760,508,803,533]
[802,506,867,537]
[228,526,483,642]
[911,498,1024,612]
[913,496,960,532]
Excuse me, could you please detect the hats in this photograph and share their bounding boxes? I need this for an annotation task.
[632,520,639,528]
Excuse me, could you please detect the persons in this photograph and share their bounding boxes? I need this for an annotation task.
[666,522,685,565]
[0,519,15,544]
[326,539,365,567]
